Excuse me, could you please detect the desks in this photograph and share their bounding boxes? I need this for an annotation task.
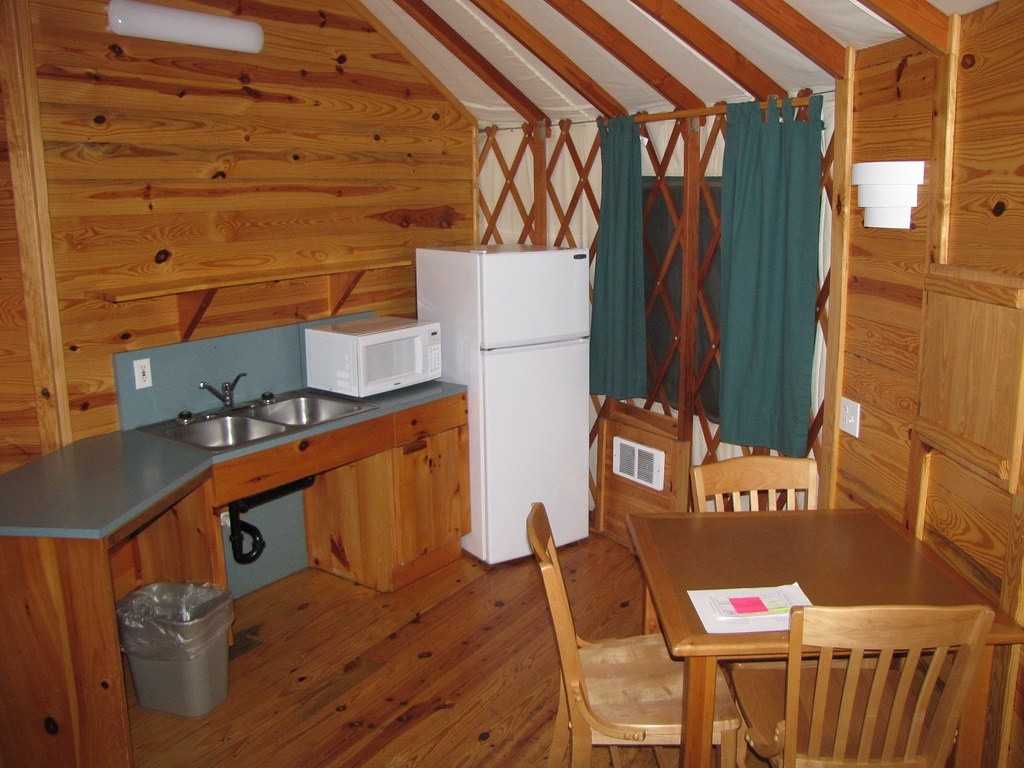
[729,605,995,768]
[623,506,1023,768]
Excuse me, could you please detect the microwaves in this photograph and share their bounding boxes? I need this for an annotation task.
[303,316,444,397]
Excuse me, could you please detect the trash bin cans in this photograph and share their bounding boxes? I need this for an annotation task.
[114,582,234,718]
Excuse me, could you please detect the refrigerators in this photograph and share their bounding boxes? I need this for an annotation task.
[416,243,591,567]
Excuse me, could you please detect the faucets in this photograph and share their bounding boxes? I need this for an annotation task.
[199,373,247,408]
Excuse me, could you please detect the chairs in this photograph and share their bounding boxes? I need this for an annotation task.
[690,454,821,512]
[525,501,742,768]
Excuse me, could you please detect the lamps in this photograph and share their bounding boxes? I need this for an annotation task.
[104,0,264,54]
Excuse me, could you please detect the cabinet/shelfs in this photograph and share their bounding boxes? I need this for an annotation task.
[0,393,473,768]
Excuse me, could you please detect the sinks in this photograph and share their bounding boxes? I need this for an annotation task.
[240,393,380,427]
[135,416,292,453]
